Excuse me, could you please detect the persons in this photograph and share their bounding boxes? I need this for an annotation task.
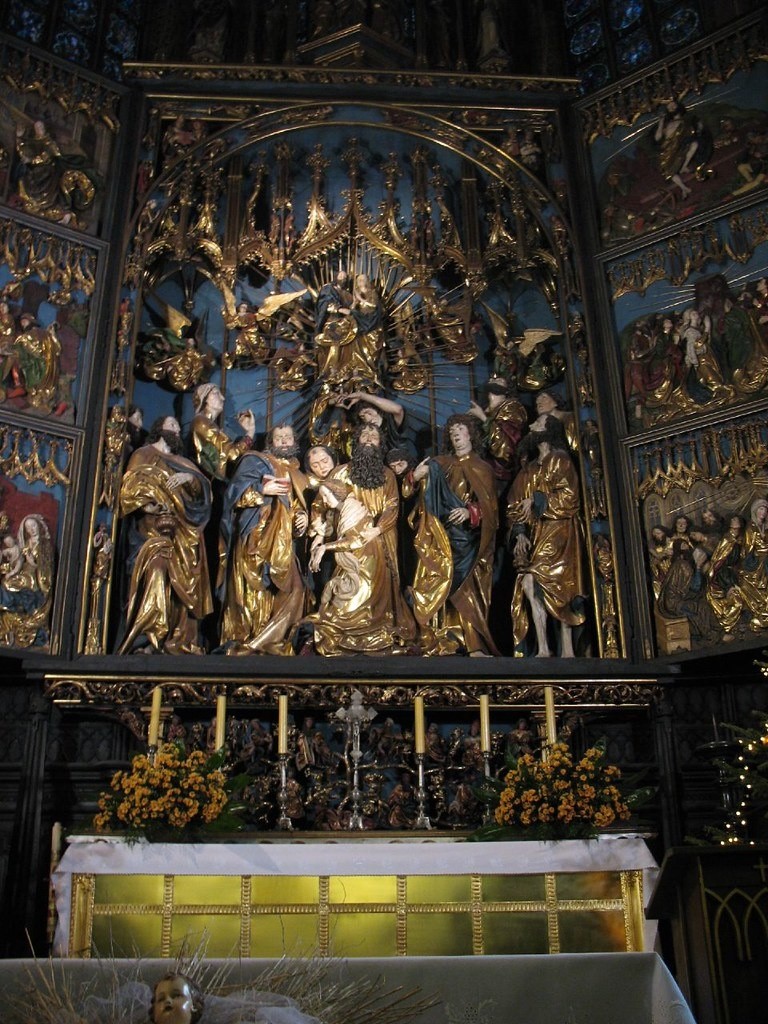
[143,716,534,832]
[0,119,768,657]
[149,974,204,1024]
[656,101,701,198]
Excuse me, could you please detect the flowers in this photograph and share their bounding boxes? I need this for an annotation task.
[451,742,633,843]
[92,736,258,846]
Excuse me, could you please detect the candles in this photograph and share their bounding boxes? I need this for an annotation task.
[480,695,491,752]
[415,697,426,754]
[545,687,557,745]
[278,696,288,754]
[215,696,226,753]
[147,685,163,746]
